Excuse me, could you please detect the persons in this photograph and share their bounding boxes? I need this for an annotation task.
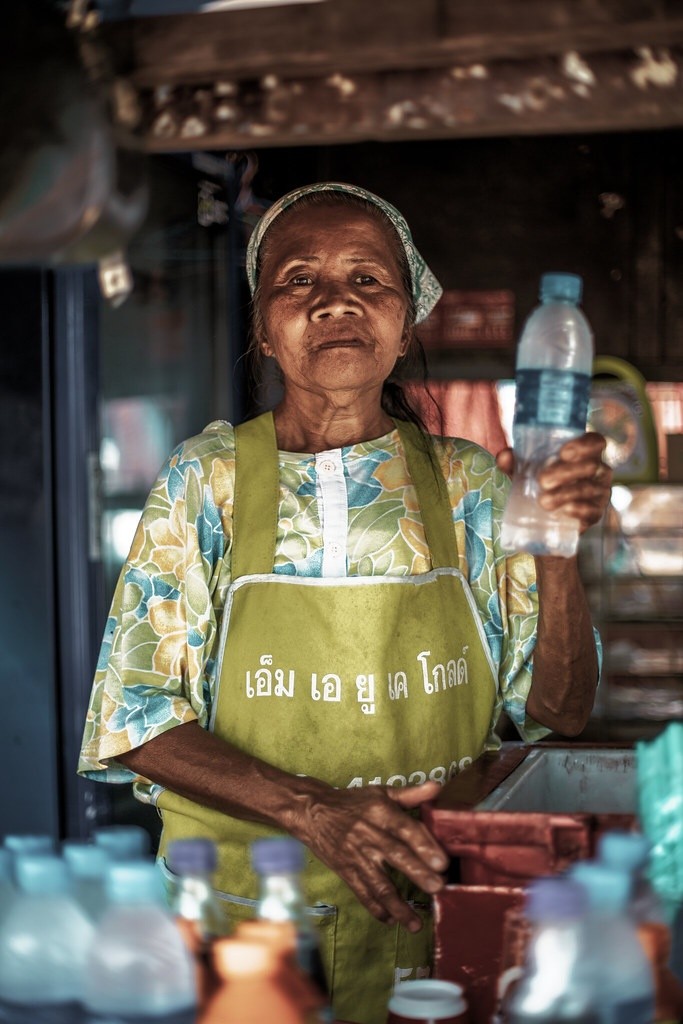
[77,181,613,1024]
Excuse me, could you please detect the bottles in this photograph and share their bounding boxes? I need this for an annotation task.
[496,273,591,560]
[0,825,339,1024]
[497,830,659,1024]
